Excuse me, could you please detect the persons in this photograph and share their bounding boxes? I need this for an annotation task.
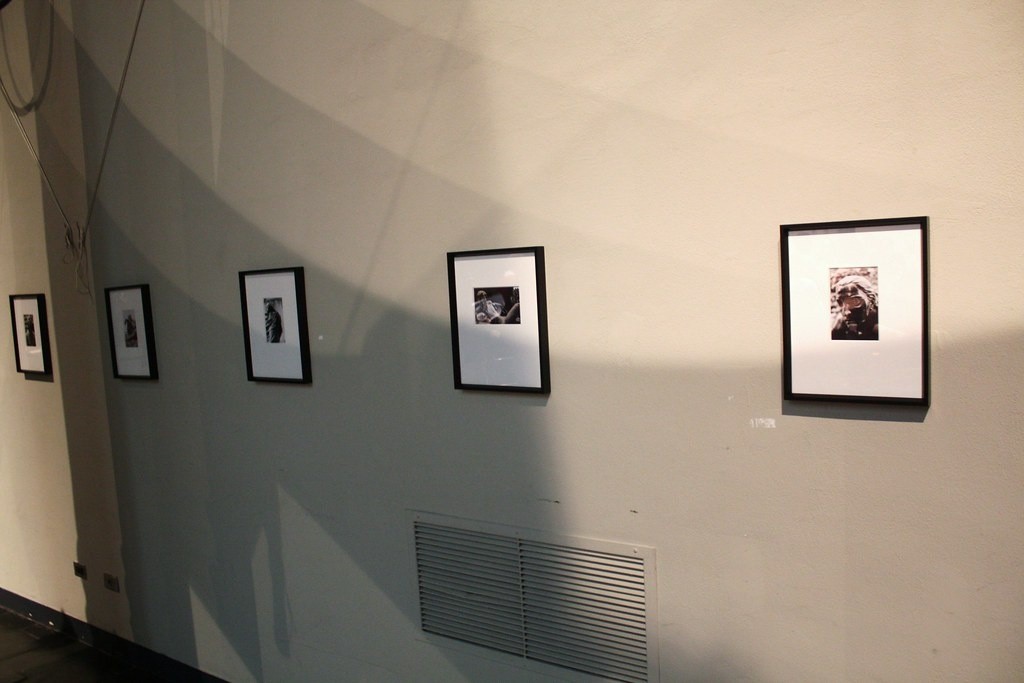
[25,316,36,346]
[125,315,136,335]
[474,288,520,323]
[265,303,282,343]
[831,277,878,340]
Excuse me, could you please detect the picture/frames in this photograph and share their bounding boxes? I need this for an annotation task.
[237,266,312,384]
[104,284,159,380]
[446,244,551,394]
[780,215,931,406]
[8,294,51,375]
